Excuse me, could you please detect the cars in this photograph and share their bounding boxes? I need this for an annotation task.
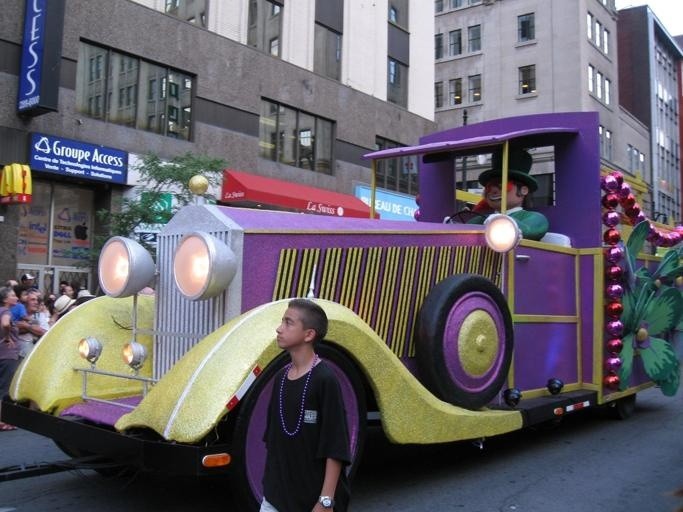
[0,111,683,512]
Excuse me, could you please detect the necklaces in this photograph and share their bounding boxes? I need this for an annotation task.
[279,355,318,436]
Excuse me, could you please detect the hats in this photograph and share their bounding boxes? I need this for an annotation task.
[21,274,35,280]
[479,142,538,193]
[54,295,77,315]
[77,290,96,299]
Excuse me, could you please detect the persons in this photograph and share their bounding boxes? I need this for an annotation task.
[0,273,98,430]
[258,300,351,512]
[461,158,550,240]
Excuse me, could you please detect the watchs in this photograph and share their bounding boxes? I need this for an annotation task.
[318,496,336,508]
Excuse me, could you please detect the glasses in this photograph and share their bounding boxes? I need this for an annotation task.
[486,181,512,194]
